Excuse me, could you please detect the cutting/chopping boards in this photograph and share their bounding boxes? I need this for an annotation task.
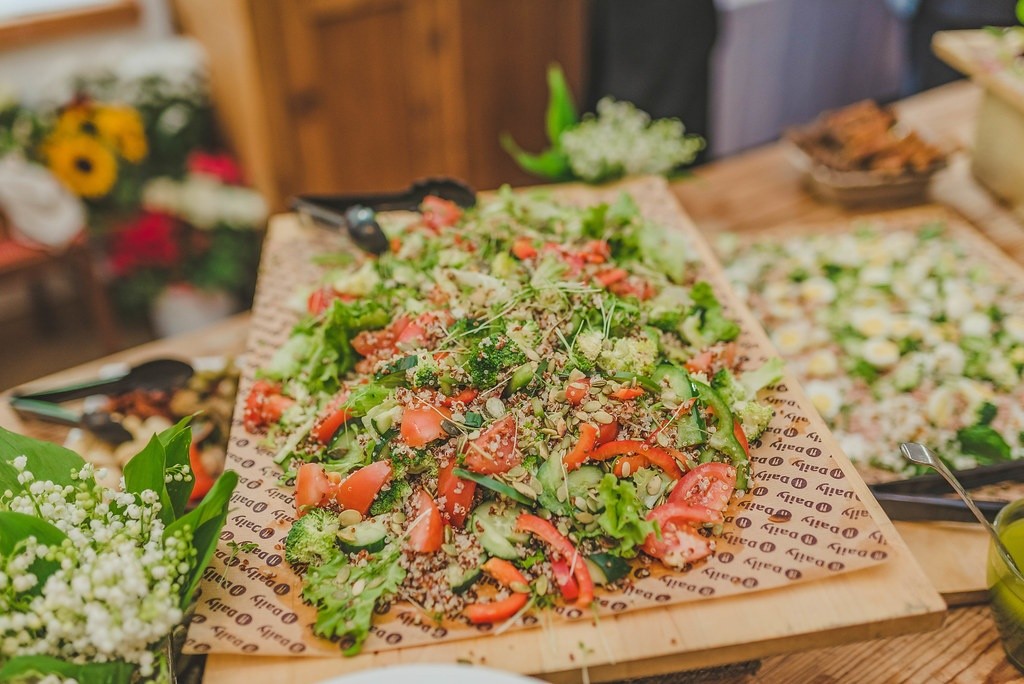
[202,173,1024,684]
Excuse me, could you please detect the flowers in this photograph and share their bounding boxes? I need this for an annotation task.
[0,81,259,286]
[504,61,703,179]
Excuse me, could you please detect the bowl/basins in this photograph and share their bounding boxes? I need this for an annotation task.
[989,500,1023,672]
[778,118,934,210]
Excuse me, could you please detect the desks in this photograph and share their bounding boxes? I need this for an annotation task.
[0,81,1024,684]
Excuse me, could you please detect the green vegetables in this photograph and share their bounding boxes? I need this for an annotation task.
[256,186,1024,655]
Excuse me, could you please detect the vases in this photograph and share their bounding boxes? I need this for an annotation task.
[147,281,235,336]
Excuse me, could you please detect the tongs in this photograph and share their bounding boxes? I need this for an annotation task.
[9,358,195,443]
[866,461,1024,524]
[288,177,476,251]
